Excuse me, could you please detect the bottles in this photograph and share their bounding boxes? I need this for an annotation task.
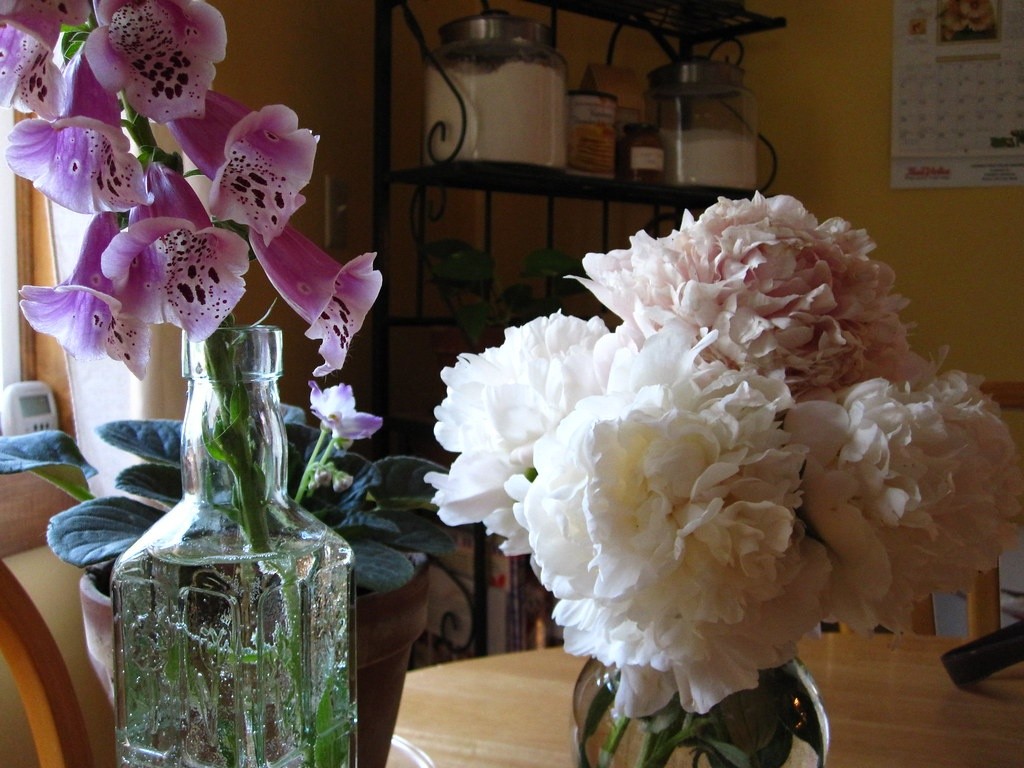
[424,14,565,168]
[654,58,757,188]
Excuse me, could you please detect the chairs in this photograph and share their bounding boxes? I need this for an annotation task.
[840,382,1024,642]
[2,472,121,767]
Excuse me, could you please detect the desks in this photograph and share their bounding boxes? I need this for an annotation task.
[385,629,1022,767]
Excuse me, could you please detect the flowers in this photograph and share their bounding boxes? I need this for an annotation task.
[1,0,450,767]
[425,191,1023,768]
[1,380,450,595]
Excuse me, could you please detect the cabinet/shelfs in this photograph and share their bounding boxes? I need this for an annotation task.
[372,0,789,666]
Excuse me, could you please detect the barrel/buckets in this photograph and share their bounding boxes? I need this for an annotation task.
[565,88,618,178]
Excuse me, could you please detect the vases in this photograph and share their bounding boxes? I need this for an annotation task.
[576,660,837,768]
[83,568,450,768]
[79,324,428,767]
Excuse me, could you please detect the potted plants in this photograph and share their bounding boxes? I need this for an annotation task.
[428,240,607,396]
